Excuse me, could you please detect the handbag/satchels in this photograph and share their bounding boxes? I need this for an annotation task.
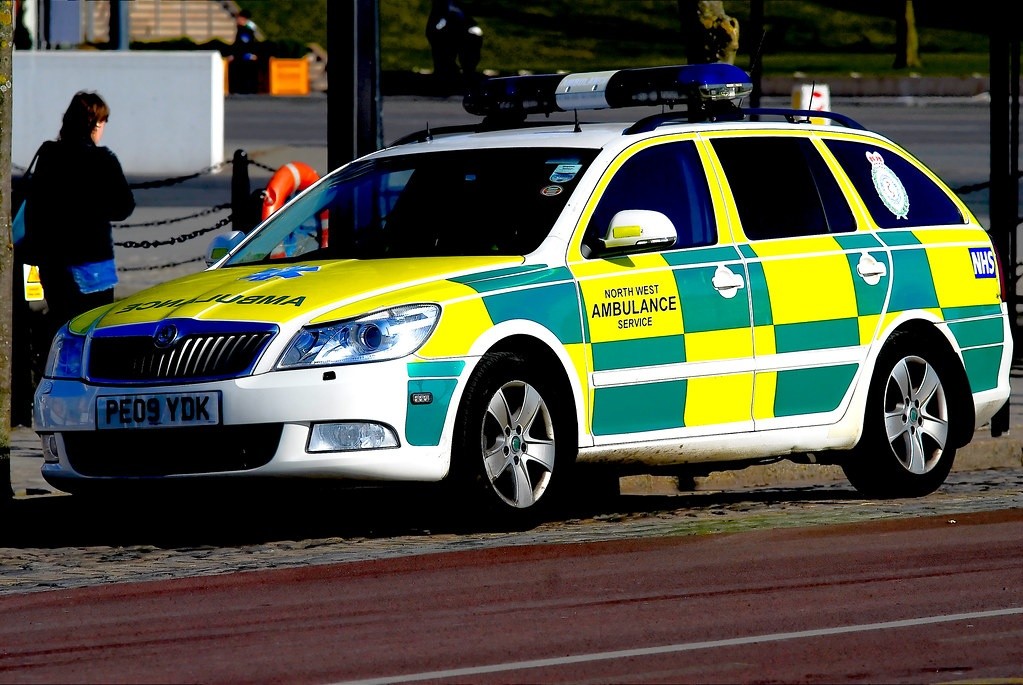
[12,142,46,264]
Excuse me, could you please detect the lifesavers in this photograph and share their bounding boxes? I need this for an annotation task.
[260,160,332,260]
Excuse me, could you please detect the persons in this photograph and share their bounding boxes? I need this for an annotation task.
[229,11,259,94]
[426,0,483,95]
[25,93,137,330]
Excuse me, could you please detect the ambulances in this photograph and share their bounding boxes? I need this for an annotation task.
[29,60,1013,532]
